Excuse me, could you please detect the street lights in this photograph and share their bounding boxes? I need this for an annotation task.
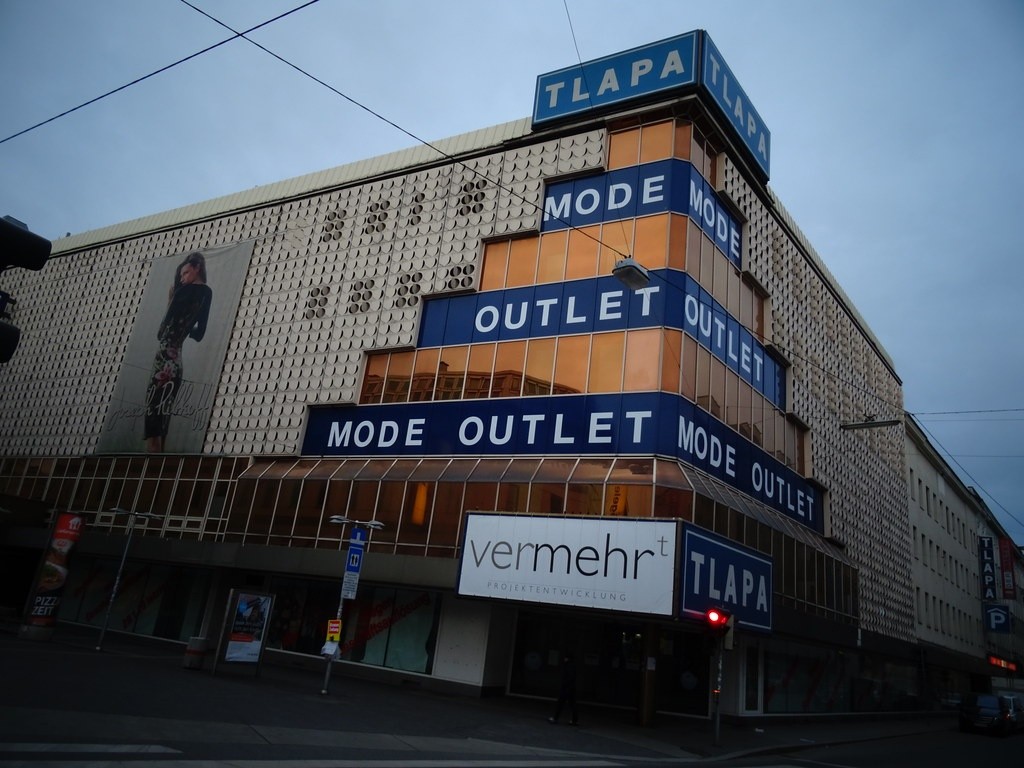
[320,510,383,699]
[91,505,165,653]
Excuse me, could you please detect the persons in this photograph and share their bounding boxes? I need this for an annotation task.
[250,606,264,640]
[548,657,578,724]
[143,254,213,452]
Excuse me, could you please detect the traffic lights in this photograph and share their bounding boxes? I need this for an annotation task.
[0,214,52,369]
[705,607,734,629]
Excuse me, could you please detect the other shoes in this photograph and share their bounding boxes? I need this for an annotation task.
[569,720,579,726]
[547,716,560,724]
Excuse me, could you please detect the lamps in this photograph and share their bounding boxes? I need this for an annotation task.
[839,419,903,430]
[612,257,651,291]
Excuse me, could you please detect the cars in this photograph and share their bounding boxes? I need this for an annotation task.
[997,692,1024,734]
[958,690,1006,736]
